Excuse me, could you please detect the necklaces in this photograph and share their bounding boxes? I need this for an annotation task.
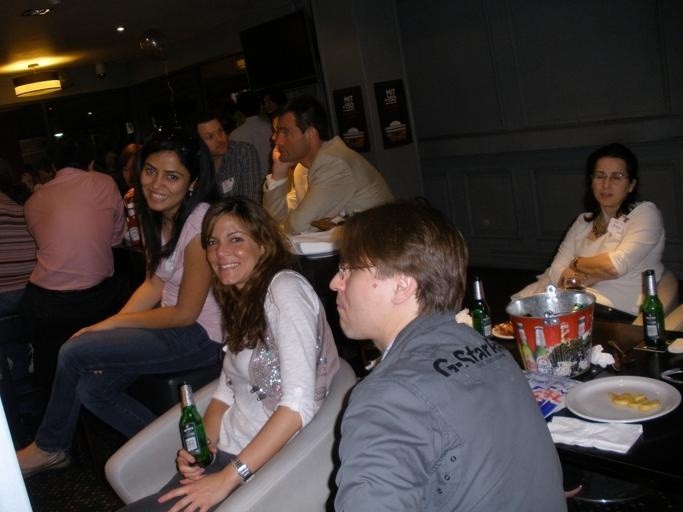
[591,213,606,238]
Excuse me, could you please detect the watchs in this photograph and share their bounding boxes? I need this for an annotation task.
[573,255,581,268]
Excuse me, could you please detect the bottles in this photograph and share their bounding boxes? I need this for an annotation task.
[578,315,586,337]
[125,202,140,245]
[534,325,551,375]
[179,381,212,468]
[641,269,666,348]
[559,323,570,342]
[516,324,535,373]
[470,278,493,342]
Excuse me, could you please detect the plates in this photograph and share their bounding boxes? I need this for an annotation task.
[565,373,682,423]
[289,243,339,260]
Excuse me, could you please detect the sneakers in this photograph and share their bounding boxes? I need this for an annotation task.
[21,448,72,479]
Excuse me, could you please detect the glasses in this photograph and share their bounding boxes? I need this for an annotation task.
[591,172,628,183]
[339,263,381,280]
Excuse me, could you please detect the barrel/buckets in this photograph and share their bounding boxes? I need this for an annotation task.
[505,284,596,378]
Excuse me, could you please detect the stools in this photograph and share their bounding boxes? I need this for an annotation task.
[0,313,51,449]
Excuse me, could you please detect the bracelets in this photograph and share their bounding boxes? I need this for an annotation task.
[229,456,254,481]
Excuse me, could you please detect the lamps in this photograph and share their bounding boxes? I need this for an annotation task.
[11,63,61,97]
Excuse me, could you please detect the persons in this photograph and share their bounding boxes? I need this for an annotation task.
[19,89,287,208]
[20,140,130,406]
[329,197,569,512]
[0,162,39,378]
[118,196,339,512]
[19,136,231,478]
[530,148,668,498]
[262,96,396,381]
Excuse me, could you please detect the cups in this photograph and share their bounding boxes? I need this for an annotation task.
[564,271,587,291]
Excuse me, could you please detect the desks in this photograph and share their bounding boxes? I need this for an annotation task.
[483,311,681,505]
